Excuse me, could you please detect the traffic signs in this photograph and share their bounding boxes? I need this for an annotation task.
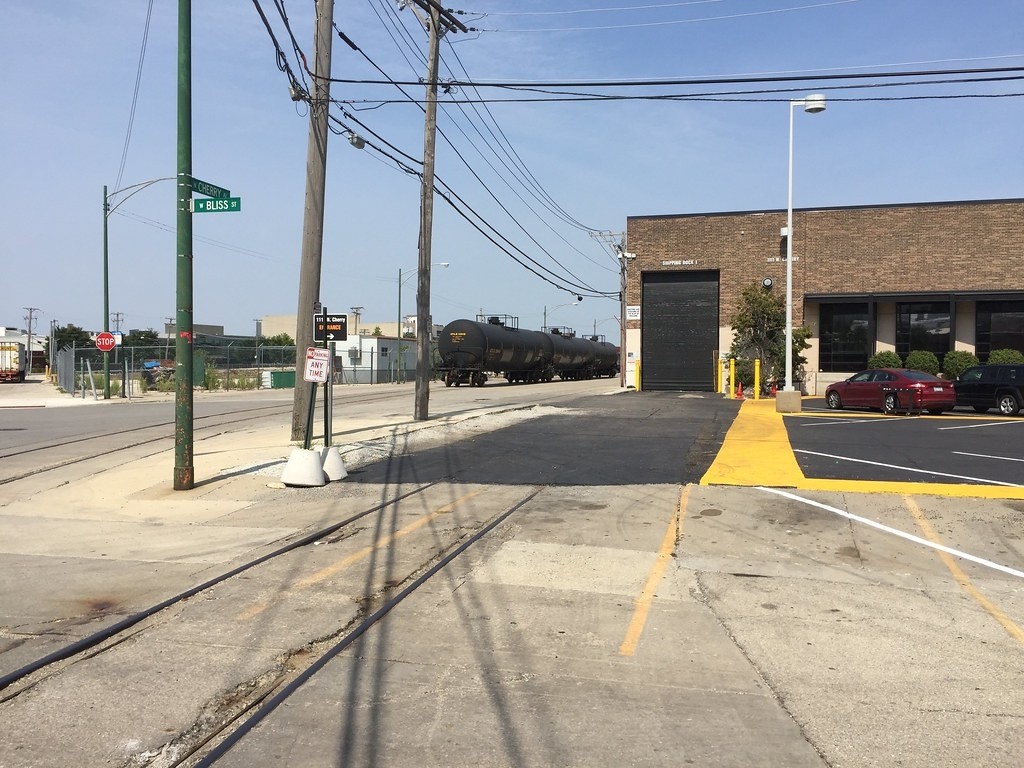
[194,197,241,213]
[192,177,230,198]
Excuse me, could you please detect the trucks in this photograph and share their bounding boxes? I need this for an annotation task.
[0,342,26,383]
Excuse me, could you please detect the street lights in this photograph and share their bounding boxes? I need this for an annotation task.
[784,93,827,387]
[398,263,449,384]
[543,303,578,333]
[594,317,621,336]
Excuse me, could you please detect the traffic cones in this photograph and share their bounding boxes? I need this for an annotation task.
[771,384,777,393]
[735,382,744,399]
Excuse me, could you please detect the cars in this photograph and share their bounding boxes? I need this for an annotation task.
[825,369,957,416]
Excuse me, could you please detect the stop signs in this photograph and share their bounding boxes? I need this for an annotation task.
[96,332,116,352]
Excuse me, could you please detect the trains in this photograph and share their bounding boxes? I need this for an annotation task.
[438,314,621,387]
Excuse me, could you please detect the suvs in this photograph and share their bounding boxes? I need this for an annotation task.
[953,363,1024,416]
